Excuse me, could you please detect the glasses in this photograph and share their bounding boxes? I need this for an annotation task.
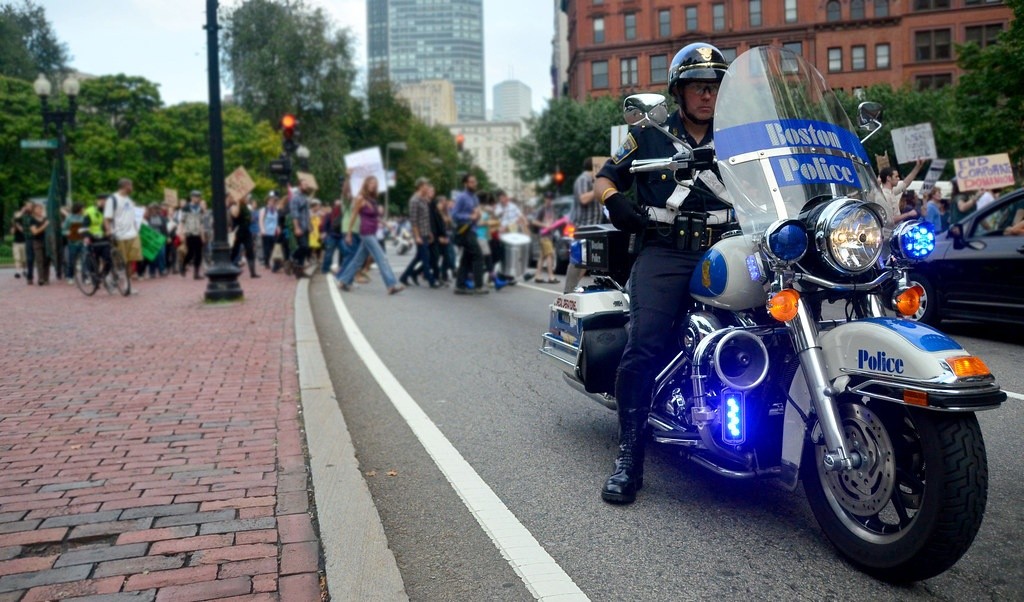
[679,81,719,96]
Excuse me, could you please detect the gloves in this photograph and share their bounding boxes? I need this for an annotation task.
[603,194,650,233]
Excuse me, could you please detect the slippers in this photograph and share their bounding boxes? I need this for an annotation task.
[534,279,547,283]
[549,278,561,284]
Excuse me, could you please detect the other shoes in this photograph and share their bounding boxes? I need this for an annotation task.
[389,285,404,294]
[400,263,519,296]
[524,272,535,281]
[12,258,340,287]
[337,282,352,292]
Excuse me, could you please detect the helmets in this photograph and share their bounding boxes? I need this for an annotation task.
[667,42,731,97]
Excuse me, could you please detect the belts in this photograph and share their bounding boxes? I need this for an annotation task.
[641,203,768,226]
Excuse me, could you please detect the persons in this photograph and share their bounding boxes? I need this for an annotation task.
[310,167,405,295]
[563,158,603,294]
[12,178,143,295]
[874,155,1024,234]
[595,42,770,505]
[225,180,315,279]
[144,191,213,280]
[474,188,561,290]
[399,174,490,294]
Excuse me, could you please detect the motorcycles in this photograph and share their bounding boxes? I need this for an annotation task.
[538,43,1008,586]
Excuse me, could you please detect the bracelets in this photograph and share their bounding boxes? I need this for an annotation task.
[602,188,616,202]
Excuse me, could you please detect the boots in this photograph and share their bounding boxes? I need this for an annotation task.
[602,368,654,503]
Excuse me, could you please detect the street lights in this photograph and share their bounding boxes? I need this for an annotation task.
[33,70,81,281]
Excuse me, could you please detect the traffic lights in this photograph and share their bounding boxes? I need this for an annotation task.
[456,134,464,151]
[280,115,301,152]
[554,171,564,183]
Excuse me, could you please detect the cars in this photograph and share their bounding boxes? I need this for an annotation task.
[890,185,1024,345]
[524,195,577,274]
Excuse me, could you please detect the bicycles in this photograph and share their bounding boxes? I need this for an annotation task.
[73,227,132,297]
